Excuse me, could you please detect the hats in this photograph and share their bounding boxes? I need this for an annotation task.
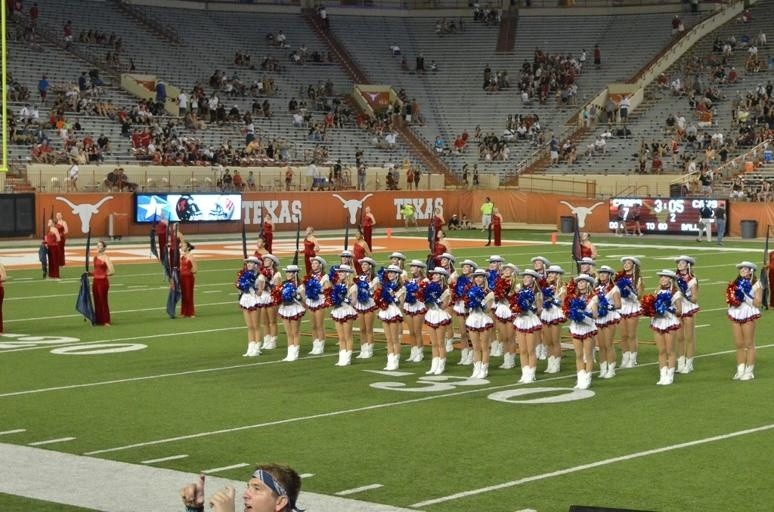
[531,255,548,264]
[485,256,505,262]
[338,251,355,258]
[596,265,615,275]
[545,265,564,274]
[309,256,326,269]
[502,262,519,274]
[656,270,676,278]
[357,256,378,268]
[388,253,406,261]
[620,256,640,265]
[437,252,456,263]
[335,265,354,273]
[577,256,596,266]
[736,261,756,271]
[471,269,490,278]
[428,266,449,276]
[384,265,404,273]
[282,265,302,271]
[675,255,695,265]
[407,260,426,267]
[575,274,595,285]
[261,254,279,266]
[244,256,262,266]
[519,269,540,278]
[459,260,478,268]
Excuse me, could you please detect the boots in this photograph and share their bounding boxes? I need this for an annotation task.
[444,339,454,352]
[455,347,469,365]
[656,366,667,385]
[307,339,325,354]
[596,361,616,380]
[733,364,745,380]
[261,336,277,349]
[384,354,400,371]
[405,346,416,361]
[583,349,597,363]
[334,350,352,367]
[544,356,560,374]
[574,368,593,390]
[413,347,423,362]
[247,341,262,356]
[740,365,754,381]
[355,343,374,359]
[681,357,694,374]
[281,345,300,362]
[478,362,489,380]
[534,344,549,361]
[463,351,474,365]
[518,367,536,384]
[499,352,516,370]
[434,357,446,375]
[661,367,675,385]
[425,356,439,373]
[676,357,686,373]
[488,340,504,356]
[470,360,482,378]
[618,351,636,369]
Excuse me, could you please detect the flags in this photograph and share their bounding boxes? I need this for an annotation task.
[166,234,181,320]
[74,237,94,325]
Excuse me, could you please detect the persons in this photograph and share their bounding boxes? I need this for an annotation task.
[87,240,116,328]
[233,253,699,390]
[181,463,302,512]
[286,0,469,254]
[473,0,643,246]
[178,240,197,318]
[45,211,180,282]
[2,0,292,190]
[724,260,763,381]
[255,212,275,257]
[0,262,11,335]
[639,1,774,246]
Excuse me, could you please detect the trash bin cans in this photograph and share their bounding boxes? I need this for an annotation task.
[764,151,772,161]
[740,220,757,238]
[560,216,573,233]
[670,184,684,199]
[744,162,753,172]
